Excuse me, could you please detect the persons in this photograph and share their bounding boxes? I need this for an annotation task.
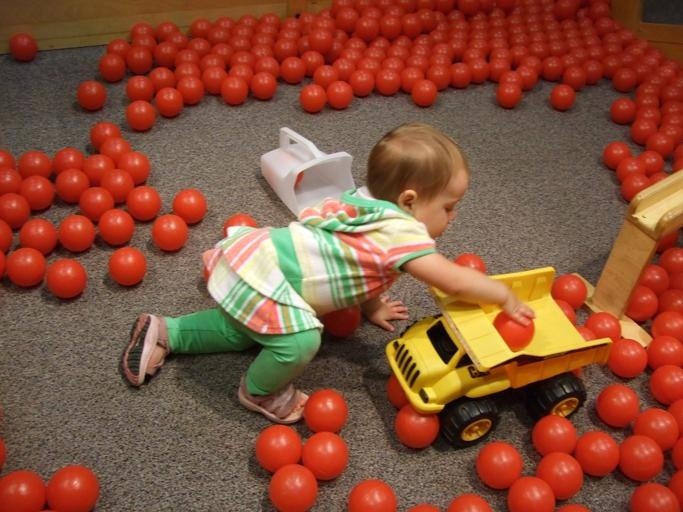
[121,122,537,425]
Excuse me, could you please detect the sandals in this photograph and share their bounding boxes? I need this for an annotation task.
[121,311,169,388]
[238,376,309,425]
